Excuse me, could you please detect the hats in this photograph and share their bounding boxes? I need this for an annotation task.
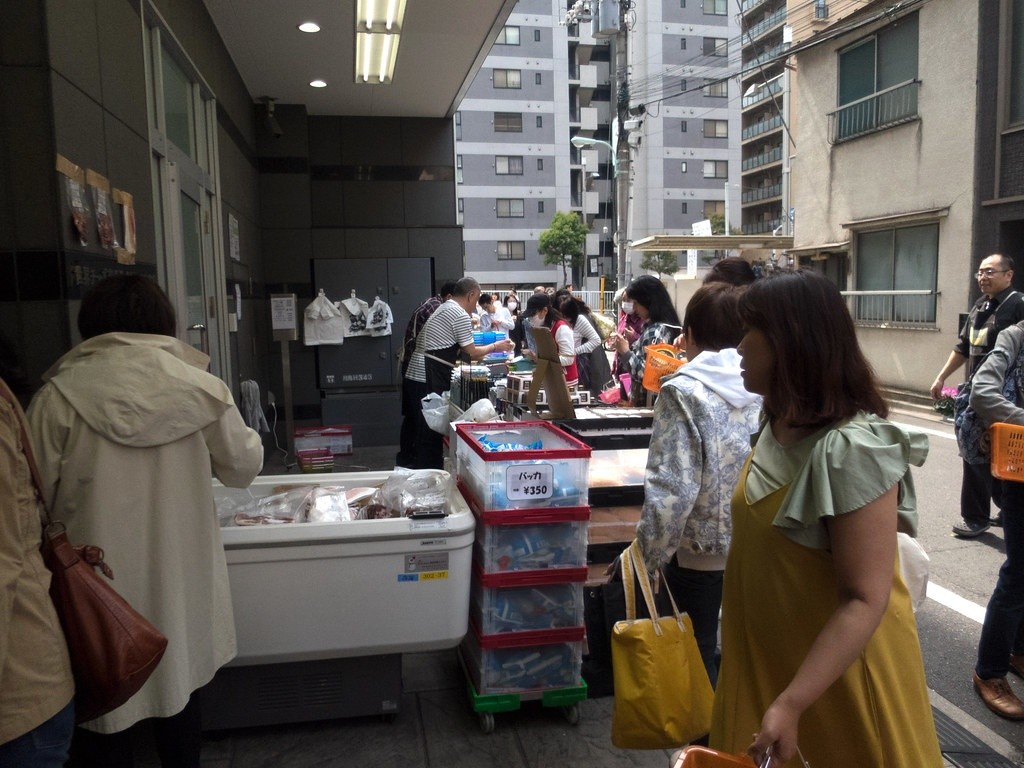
[522,294,549,314]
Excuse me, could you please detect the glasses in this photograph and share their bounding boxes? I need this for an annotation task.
[974,269,1007,279]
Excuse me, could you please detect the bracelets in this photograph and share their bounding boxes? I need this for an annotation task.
[493,343,496,352]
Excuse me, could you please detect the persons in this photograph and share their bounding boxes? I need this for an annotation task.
[397,276,512,470]
[968,295,1024,720]
[706,269,944,768]
[603,282,766,750]
[0,377,77,768]
[478,257,760,408]
[25,272,265,768]
[928,254,1024,538]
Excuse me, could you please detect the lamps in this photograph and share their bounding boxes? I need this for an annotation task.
[353,0,406,83]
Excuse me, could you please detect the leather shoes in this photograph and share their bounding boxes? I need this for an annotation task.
[1009,654,1024,679]
[973,673,1024,720]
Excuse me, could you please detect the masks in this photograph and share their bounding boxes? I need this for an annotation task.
[528,315,545,326]
[508,302,517,311]
[621,302,635,315]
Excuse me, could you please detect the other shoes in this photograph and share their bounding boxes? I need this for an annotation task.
[953,518,990,537]
[988,511,1003,527]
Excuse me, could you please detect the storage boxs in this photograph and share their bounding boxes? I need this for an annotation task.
[454,417,655,713]
[294,426,354,474]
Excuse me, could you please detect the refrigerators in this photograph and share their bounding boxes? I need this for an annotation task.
[199,469,477,734]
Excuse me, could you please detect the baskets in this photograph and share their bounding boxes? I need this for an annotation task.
[642,344,686,394]
[989,422,1024,482]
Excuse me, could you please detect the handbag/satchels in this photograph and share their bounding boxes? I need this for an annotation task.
[610,538,716,751]
[954,346,1024,465]
[43,520,168,726]
[589,311,615,339]
